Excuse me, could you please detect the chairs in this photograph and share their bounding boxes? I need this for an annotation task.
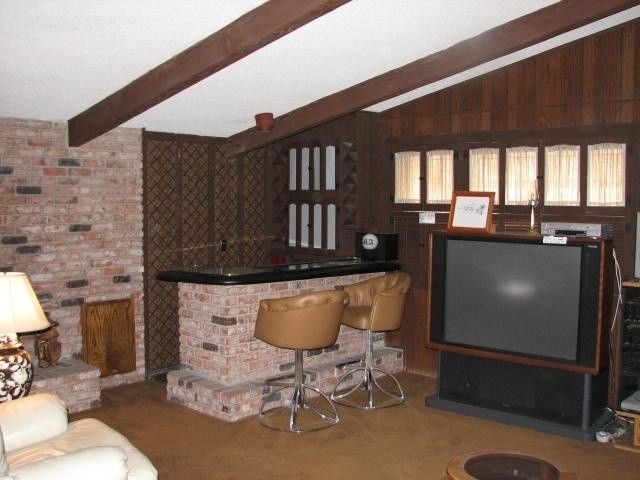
[0,392,159,480]
[254,271,411,436]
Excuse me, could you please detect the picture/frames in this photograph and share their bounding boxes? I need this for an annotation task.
[447,190,496,234]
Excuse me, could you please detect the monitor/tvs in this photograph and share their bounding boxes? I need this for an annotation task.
[431,232,602,370]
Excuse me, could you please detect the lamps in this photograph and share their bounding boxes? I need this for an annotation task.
[0,271,52,404]
